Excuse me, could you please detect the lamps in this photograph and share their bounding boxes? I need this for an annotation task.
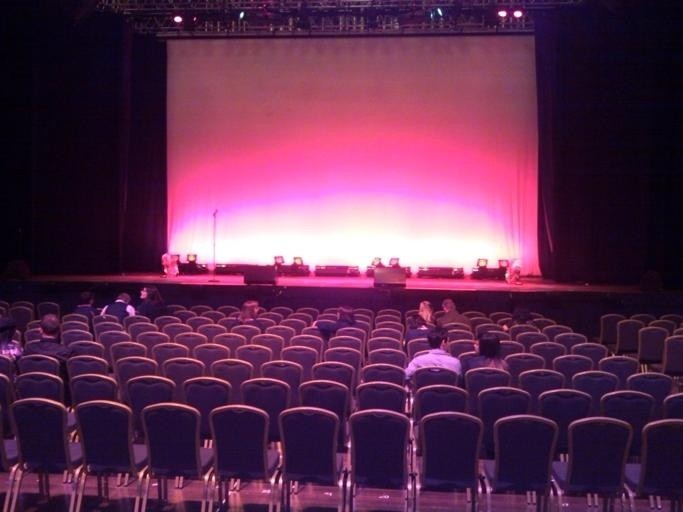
[496,6,525,18]
[294,257,303,266]
[477,258,488,267]
[274,255,285,265]
[171,9,248,22]
[429,6,448,18]
[389,258,399,265]
[371,257,381,266]
[187,253,198,263]
[499,259,509,268]
[172,254,180,262]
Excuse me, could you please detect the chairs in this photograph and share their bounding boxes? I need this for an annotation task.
[0,304,683,509]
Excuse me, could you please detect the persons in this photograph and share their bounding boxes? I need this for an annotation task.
[0,314,75,403]
[70,291,98,330]
[230,299,266,334]
[311,305,368,334]
[98,284,169,329]
[404,298,534,381]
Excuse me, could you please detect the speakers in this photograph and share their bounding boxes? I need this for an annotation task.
[374,269,406,288]
[243,269,275,284]
